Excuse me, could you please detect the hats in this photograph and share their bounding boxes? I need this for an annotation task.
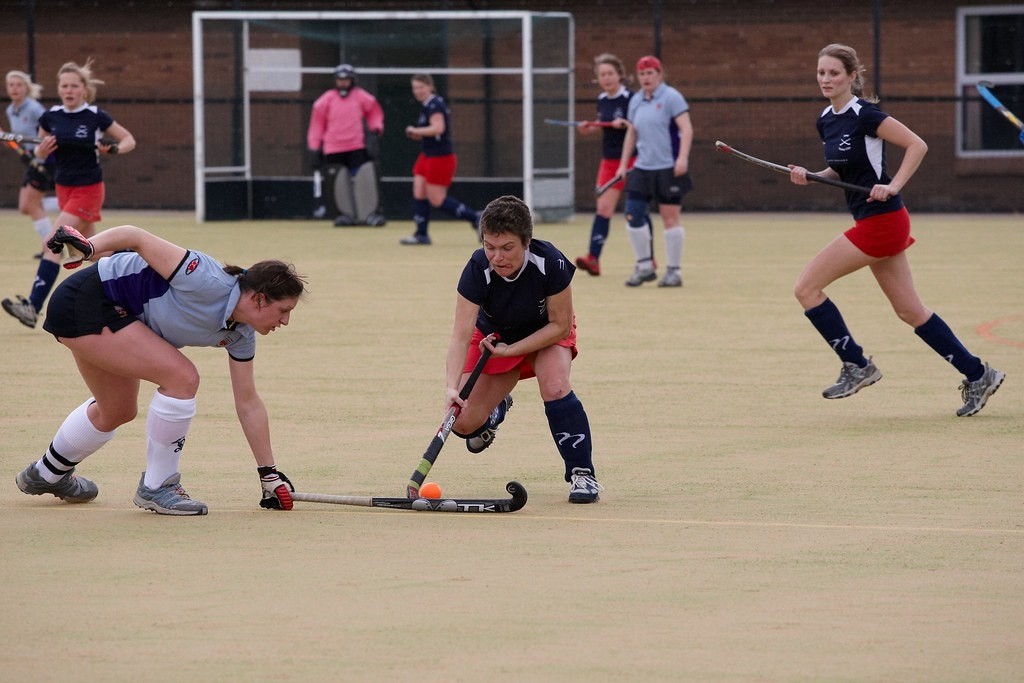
[638,57,660,69]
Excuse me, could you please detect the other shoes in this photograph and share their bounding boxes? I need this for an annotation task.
[577,254,600,275]
[626,266,657,288]
[659,269,682,285]
[398,235,429,244]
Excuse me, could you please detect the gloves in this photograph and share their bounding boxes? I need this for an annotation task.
[47,225,94,261]
[258,464,294,511]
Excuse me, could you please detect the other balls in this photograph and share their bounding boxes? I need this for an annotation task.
[418,481,442,500]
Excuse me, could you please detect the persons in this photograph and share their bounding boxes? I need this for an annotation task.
[2,61,135,328]
[13,224,294,514]
[399,73,485,244]
[444,196,603,503]
[6,71,60,258]
[307,64,386,227]
[616,56,694,287]
[576,53,657,276]
[788,44,1006,417]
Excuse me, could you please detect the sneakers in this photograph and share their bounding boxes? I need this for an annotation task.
[16,461,97,502]
[131,472,208,515]
[955,363,1006,417]
[0,295,38,328]
[821,355,882,399]
[467,394,512,453]
[569,467,604,502]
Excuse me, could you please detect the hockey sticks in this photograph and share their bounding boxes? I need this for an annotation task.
[715,139,892,201]
[0,128,54,183]
[405,333,502,498]
[309,170,326,219]
[288,479,529,515]
[544,119,626,129]
[591,168,631,199]
[0,132,119,155]
[975,79,1024,131]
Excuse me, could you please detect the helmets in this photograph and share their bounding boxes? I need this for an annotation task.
[333,65,356,95]
